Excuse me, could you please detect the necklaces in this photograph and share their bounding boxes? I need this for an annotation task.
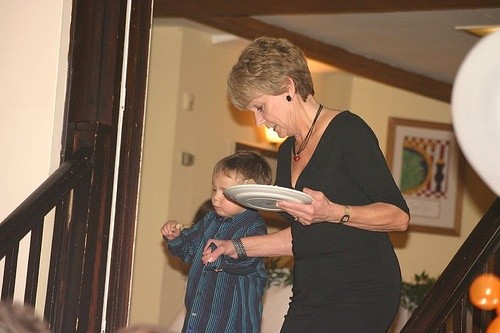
[293,104,323,162]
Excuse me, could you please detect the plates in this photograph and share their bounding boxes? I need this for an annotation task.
[224,186,313,211]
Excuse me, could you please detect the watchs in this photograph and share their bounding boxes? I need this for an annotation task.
[339,205,350,225]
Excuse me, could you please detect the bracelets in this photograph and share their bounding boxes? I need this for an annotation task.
[231,237,247,260]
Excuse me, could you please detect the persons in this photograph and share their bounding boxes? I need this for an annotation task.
[204,36,411,333]
[161,150,272,333]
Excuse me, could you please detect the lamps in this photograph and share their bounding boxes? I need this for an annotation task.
[265,126,287,147]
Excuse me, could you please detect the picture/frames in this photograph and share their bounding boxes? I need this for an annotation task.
[385,116,462,237]
